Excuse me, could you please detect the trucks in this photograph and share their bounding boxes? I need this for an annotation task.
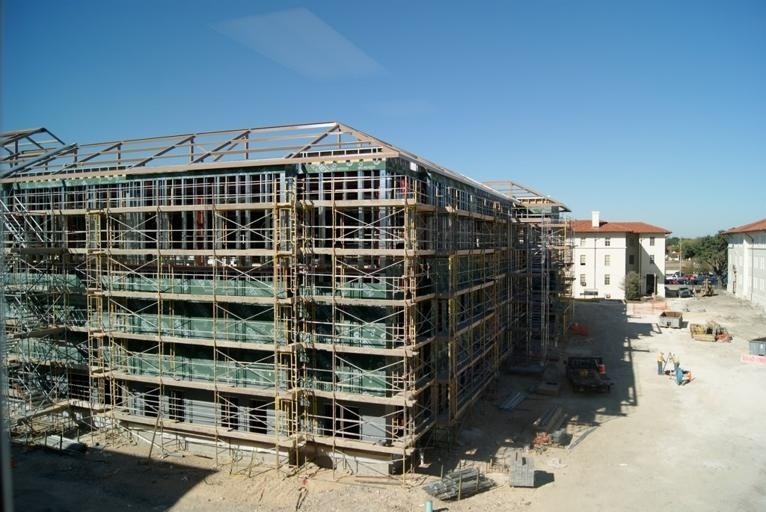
[563,355,614,394]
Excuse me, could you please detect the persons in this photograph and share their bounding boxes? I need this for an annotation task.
[657,351,686,385]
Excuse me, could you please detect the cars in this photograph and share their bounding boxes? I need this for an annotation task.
[676,287,692,297]
[666,271,715,285]
